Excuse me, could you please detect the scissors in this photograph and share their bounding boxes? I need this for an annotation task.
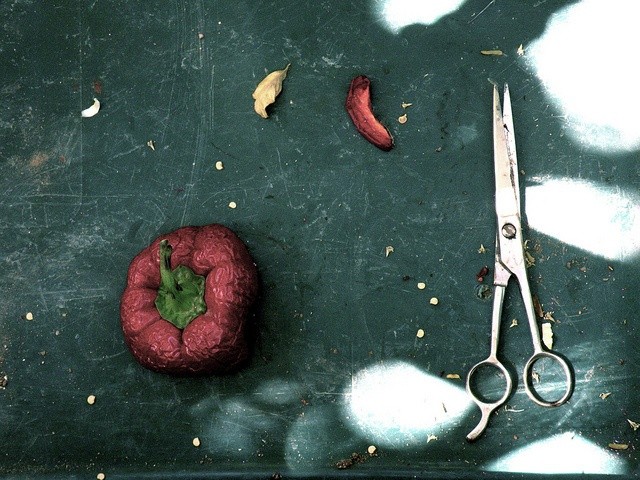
[466,83,575,442]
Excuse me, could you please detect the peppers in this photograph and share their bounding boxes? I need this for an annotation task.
[347,74,394,150]
